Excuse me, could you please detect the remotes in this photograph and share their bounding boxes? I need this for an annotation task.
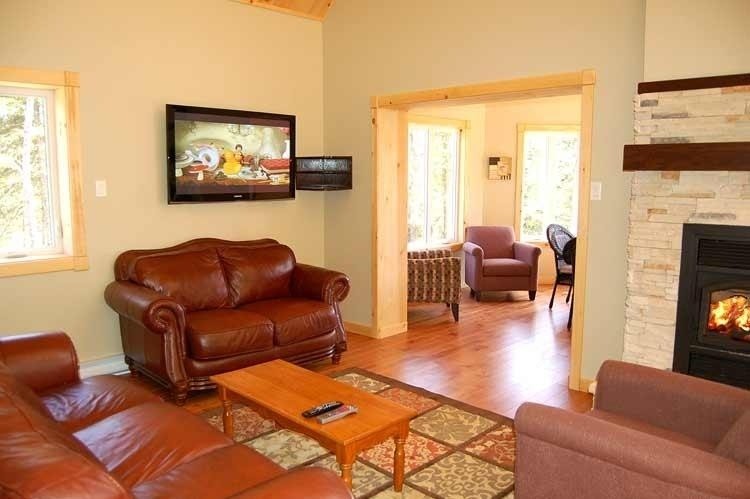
[316,403,358,424]
[303,400,344,418]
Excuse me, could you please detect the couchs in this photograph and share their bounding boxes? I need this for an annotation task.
[463,226,541,301]
[105,239,348,397]
[515,358,750,499]
[0,331,353,499]
[405,250,461,322]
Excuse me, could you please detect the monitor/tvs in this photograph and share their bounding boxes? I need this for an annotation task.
[166,103,297,203]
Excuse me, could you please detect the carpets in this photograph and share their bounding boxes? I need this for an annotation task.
[193,366,515,499]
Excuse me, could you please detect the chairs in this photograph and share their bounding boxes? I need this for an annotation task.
[547,223,577,329]
